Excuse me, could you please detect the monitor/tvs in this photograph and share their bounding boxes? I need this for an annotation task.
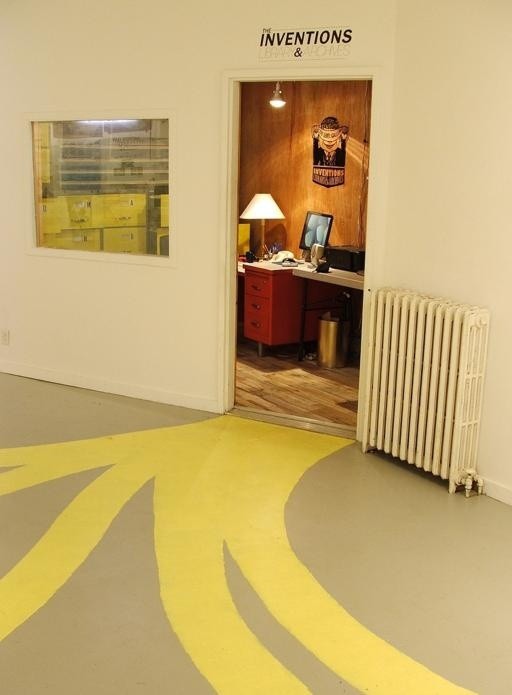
[299,211,333,262]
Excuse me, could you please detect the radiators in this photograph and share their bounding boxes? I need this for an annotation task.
[362,288,484,498]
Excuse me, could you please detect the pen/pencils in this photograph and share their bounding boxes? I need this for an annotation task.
[263,243,281,253]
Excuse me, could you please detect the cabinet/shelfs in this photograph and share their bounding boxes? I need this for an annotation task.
[242,264,336,357]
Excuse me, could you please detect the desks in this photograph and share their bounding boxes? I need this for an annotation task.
[293,267,363,361]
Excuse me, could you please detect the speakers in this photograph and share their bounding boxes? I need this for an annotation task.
[307,244,325,268]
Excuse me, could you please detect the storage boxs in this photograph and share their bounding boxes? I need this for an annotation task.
[42,193,148,254]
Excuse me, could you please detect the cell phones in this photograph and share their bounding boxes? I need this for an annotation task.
[282,262,298,267]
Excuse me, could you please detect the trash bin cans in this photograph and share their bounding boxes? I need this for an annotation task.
[318,318,349,369]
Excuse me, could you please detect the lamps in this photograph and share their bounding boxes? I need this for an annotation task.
[270,82,286,107]
[240,194,286,259]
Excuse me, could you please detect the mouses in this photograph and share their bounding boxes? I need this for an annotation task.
[282,258,296,262]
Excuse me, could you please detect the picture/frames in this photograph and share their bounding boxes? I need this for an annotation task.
[300,212,333,249]
